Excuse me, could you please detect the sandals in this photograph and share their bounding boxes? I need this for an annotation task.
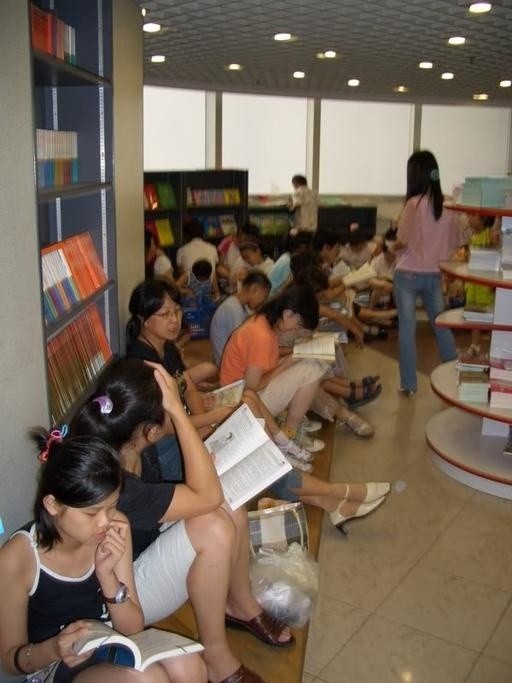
[350,375,380,388]
[338,413,375,438]
[343,383,384,410]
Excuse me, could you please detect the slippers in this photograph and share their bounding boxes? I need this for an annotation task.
[224,607,297,647]
[217,663,267,683]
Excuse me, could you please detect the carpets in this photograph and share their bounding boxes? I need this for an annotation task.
[144,325,359,682]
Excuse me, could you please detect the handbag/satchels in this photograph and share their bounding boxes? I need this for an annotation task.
[241,501,310,561]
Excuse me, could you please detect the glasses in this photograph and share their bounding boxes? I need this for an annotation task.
[152,307,184,319]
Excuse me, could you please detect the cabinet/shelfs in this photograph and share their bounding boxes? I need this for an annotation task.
[244,203,378,256]
[27,0,117,432]
[423,200,511,496]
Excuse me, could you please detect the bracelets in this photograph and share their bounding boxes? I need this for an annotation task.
[13,643,35,674]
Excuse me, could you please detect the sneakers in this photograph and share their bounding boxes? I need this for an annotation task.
[278,440,314,474]
[280,423,326,452]
[276,408,322,434]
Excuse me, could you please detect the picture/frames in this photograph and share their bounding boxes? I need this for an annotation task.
[144,169,249,262]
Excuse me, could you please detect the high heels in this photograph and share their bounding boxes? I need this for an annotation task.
[397,386,416,395]
[343,481,391,503]
[328,496,387,537]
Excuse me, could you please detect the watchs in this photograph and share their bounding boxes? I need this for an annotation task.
[97,583,129,604]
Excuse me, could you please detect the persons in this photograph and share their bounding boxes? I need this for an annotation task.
[288,176,317,249]
[144,222,397,473]
[441,213,503,361]
[124,278,391,536]
[391,151,458,393]
[68,353,295,683]
[2,425,210,683]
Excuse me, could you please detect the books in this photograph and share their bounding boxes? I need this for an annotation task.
[35,128,78,187]
[205,402,292,511]
[28,3,84,65]
[39,230,115,426]
[71,620,205,672]
[144,181,241,246]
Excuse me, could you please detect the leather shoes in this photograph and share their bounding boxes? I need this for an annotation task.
[363,326,388,340]
